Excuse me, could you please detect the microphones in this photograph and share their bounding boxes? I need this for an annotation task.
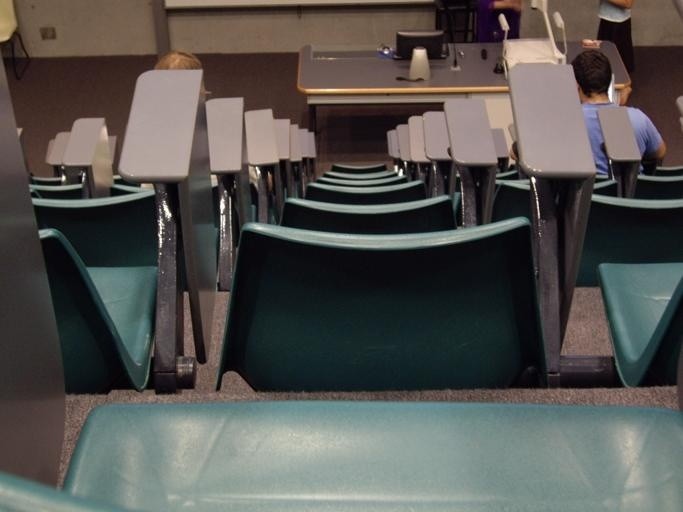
[445,7,458,67]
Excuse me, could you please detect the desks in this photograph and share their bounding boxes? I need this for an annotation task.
[297,40,632,132]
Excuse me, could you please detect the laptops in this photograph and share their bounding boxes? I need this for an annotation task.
[393,29,447,59]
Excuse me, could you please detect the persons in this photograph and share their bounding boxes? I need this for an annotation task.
[510,49,667,178]
[473,0,522,43]
[594,0,636,74]
[140,50,274,193]
